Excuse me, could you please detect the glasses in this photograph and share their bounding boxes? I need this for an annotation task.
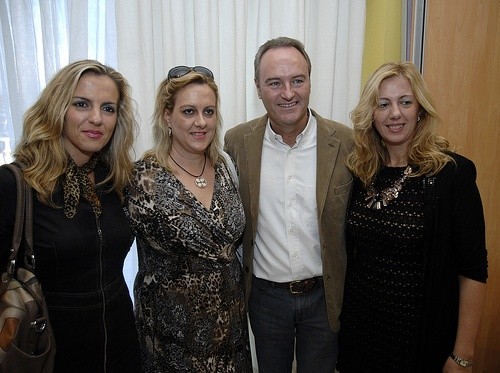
[168,65,215,81]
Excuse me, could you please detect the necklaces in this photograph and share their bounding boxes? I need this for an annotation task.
[167,152,208,189]
[362,165,414,210]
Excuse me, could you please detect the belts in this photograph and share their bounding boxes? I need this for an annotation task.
[274,277,320,293]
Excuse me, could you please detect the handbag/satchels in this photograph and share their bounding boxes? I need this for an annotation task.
[0,164,57,373]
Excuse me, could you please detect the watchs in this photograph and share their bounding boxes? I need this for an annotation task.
[449,353,473,369]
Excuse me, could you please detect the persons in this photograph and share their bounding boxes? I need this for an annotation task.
[224,36,353,373]
[344,61,489,373]
[0,59,142,373]
[119,66,254,373]
